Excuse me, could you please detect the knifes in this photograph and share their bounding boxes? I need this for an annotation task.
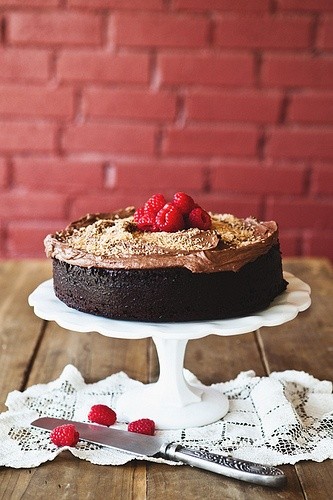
[30,416,288,488]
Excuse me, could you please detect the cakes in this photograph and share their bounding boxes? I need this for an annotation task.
[44,192,289,323]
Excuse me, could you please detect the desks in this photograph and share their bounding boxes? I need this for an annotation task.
[0,257,333,499]
